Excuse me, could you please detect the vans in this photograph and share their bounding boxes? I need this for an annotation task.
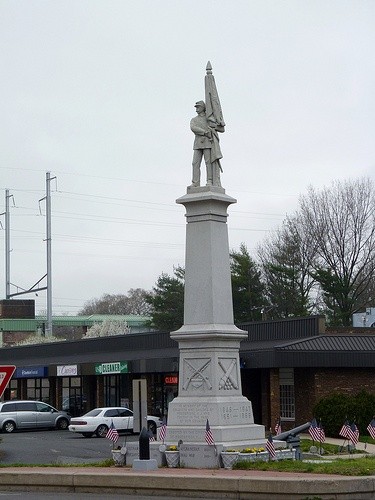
[0,400,72,432]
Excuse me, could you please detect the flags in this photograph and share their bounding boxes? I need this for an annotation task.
[339,419,351,440]
[316,419,326,443]
[265,430,276,457]
[307,418,319,442]
[147,427,155,442]
[159,416,167,440]
[105,422,122,445]
[204,418,214,445]
[274,415,282,435]
[202,73,226,165]
[347,420,360,445]
[367,418,375,440]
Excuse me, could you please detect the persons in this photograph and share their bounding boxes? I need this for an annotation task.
[188,99,216,188]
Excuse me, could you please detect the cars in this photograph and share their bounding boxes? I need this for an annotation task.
[68,406,161,438]
